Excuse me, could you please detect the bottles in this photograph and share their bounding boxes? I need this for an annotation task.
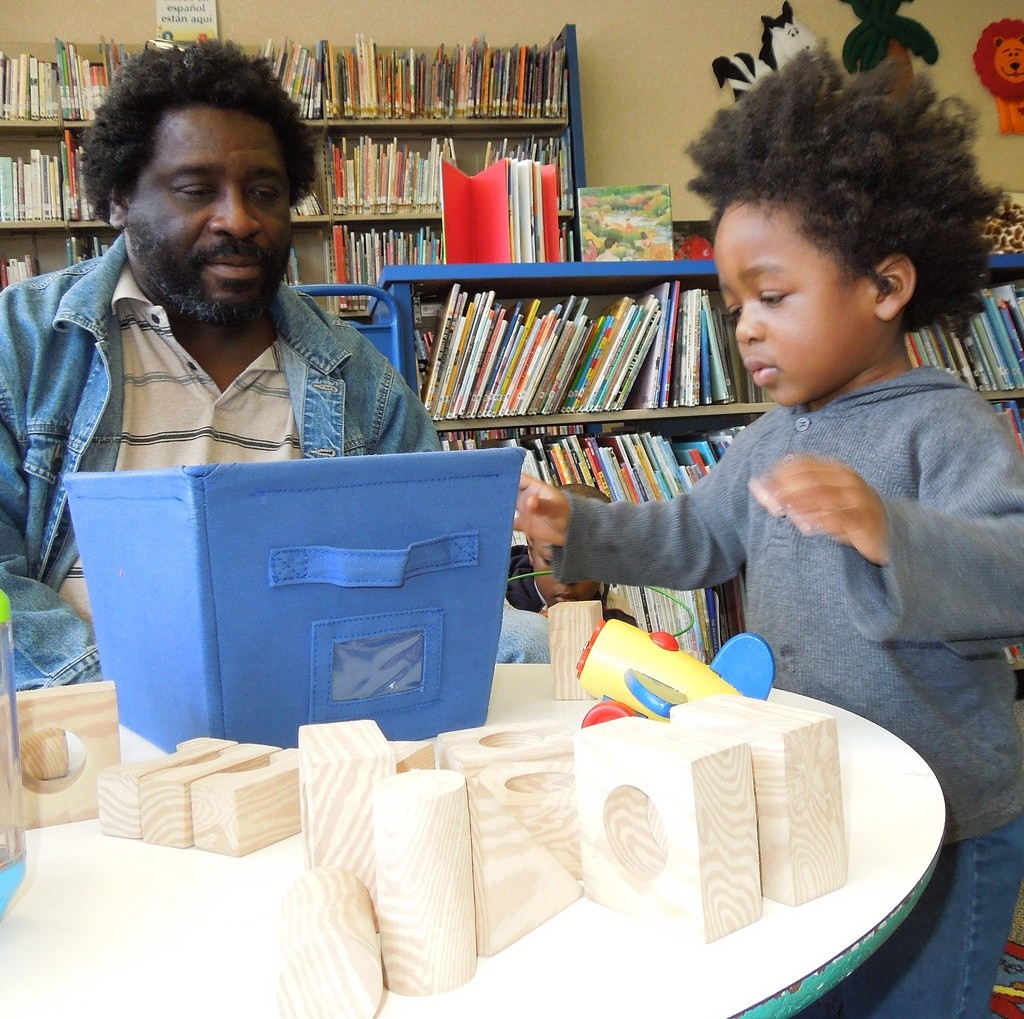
[1,588,26,920]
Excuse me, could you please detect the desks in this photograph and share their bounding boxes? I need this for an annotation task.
[4,651,951,1017]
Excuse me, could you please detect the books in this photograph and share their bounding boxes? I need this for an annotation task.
[423,280,1024,660]
[0,43,579,314]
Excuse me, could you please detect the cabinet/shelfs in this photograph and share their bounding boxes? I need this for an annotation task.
[376,263,1023,696]
[0,26,583,335]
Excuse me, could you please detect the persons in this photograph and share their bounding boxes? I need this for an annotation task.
[513,38,1024,1019]
[1,32,547,692]
